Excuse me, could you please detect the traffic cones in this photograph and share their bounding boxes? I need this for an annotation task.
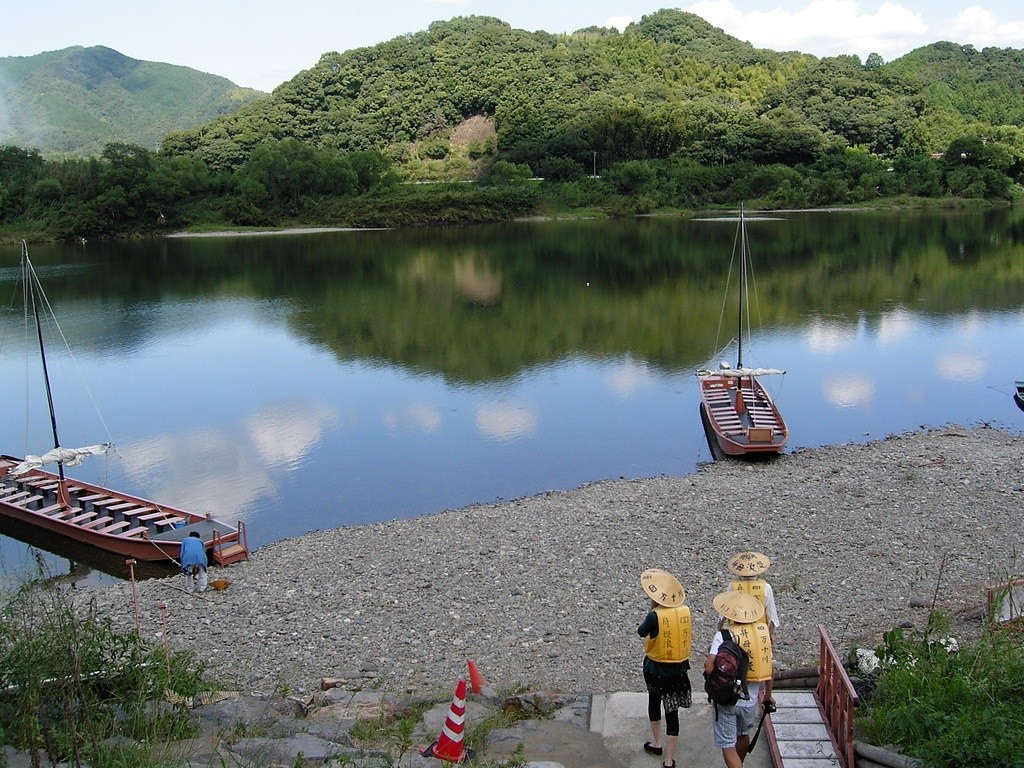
[465,658,489,700]
[418,678,476,766]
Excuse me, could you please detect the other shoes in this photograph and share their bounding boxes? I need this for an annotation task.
[663,760,676,768]
[644,741,663,755]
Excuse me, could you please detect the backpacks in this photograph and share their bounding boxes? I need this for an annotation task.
[703,628,750,721]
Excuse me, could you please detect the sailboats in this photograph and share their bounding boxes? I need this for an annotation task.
[693,200,791,457]
[0,231,251,570]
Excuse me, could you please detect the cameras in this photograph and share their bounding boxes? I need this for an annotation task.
[762,700,776,713]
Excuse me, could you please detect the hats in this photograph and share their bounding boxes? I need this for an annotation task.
[728,552,770,576]
[714,591,765,623]
[210,580,231,590]
[640,568,685,607]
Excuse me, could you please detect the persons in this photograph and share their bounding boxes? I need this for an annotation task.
[717,553,781,654]
[637,569,693,768]
[703,592,776,768]
[180,531,208,594]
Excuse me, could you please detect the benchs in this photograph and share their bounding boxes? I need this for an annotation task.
[0,475,185,538]
[705,387,784,435]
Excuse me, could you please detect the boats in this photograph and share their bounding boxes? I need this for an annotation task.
[1014,380,1024,404]
